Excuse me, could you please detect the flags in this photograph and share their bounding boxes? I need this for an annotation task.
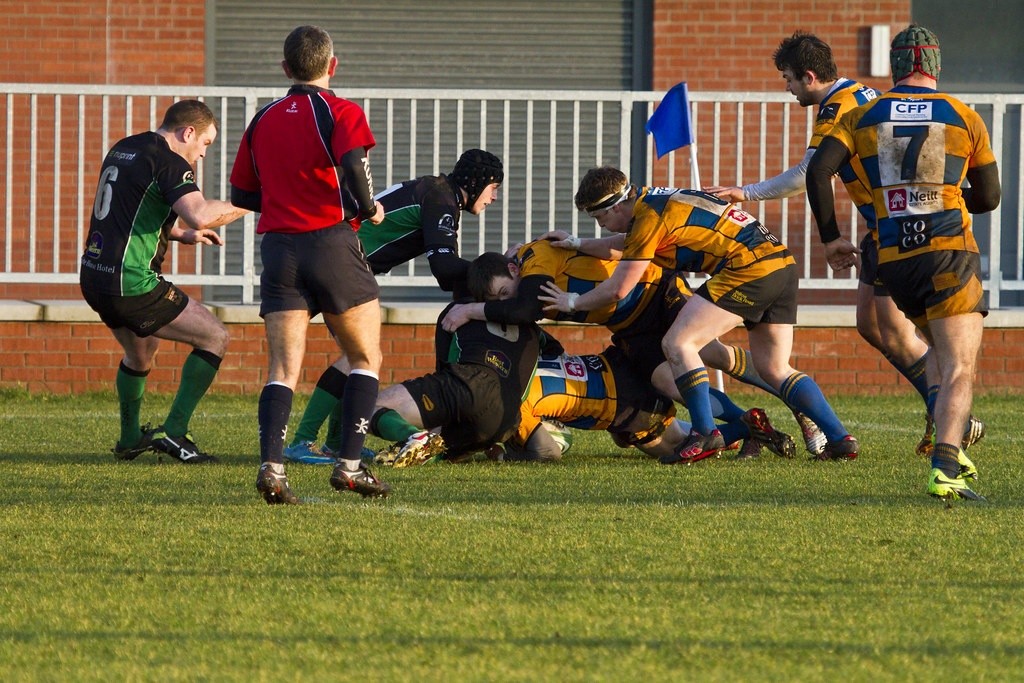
[646,82,691,161]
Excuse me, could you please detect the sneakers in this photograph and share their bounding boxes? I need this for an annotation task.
[809,435,859,461]
[927,468,987,502]
[331,462,388,497]
[151,426,218,464]
[114,422,154,460]
[962,413,985,451]
[737,438,764,459]
[658,428,726,463]
[284,441,336,464]
[392,429,445,468]
[958,445,978,487]
[916,412,936,458]
[320,443,375,457]
[373,440,405,463]
[741,408,796,460]
[794,407,828,455]
[257,464,297,505]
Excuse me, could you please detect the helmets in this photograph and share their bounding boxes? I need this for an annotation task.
[454,148,505,211]
[890,26,941,83]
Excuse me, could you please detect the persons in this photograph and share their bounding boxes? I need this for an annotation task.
[806,25,1001,501]
[280,149,524,464]
[364,269,565,467]
[441,240,827,455]
[537,166,859,461]
[487,345,796,459]
[703,30,986,455]
[229,26,394,505]
[80,99,250,466]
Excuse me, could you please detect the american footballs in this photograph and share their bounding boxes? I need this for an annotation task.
[541,420,572,456]
